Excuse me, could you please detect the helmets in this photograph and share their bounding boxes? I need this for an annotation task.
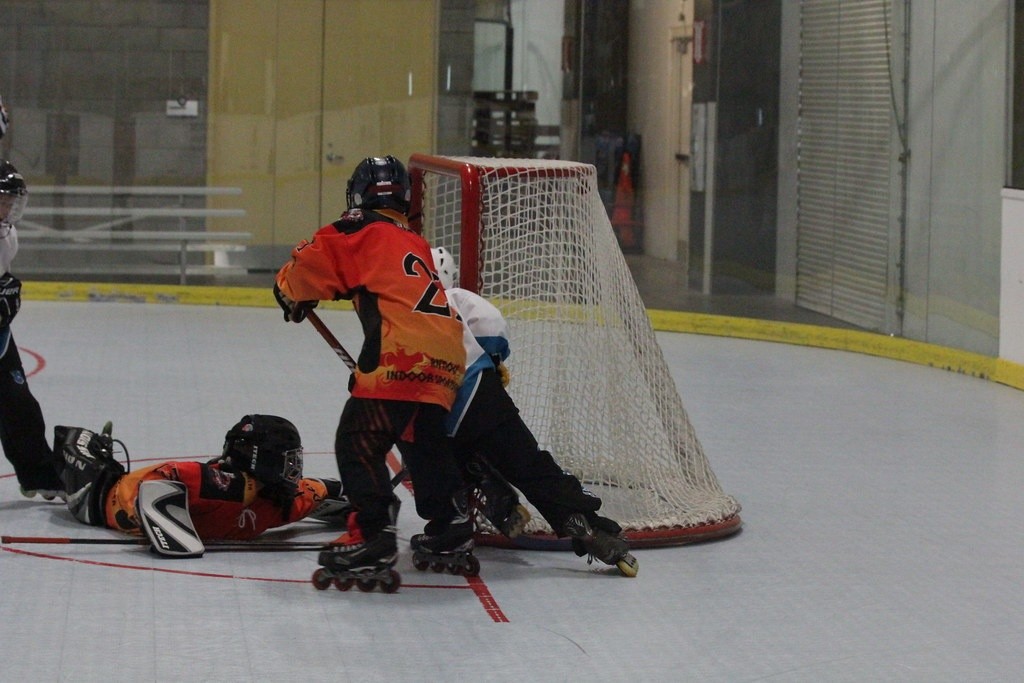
[0,160,29,226]
[345,154,410,215]
[430,246,456,289]
[223,413,304,503]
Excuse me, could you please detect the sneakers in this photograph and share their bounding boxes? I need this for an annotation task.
[410,531,480,578]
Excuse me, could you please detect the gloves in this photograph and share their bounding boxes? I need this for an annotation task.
[499,359,510,389]
[0,271,22,324]
[274,284,320,324]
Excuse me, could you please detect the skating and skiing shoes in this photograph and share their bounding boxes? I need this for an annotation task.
[19,453,72,502]
[566,511,640,577]
[312,541,401,593]
[483,485,529,538]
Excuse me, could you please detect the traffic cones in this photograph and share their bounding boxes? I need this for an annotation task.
[607,152,649,256]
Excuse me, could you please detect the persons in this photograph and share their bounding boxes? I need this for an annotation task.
[54,413,344,557]
[273,155,479,594]
[420,246,642,579]
[0,159,113,503]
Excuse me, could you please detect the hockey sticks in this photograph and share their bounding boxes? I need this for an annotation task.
[1,534,329,546]
[391,466,410,491]
[306,309,358,372]
